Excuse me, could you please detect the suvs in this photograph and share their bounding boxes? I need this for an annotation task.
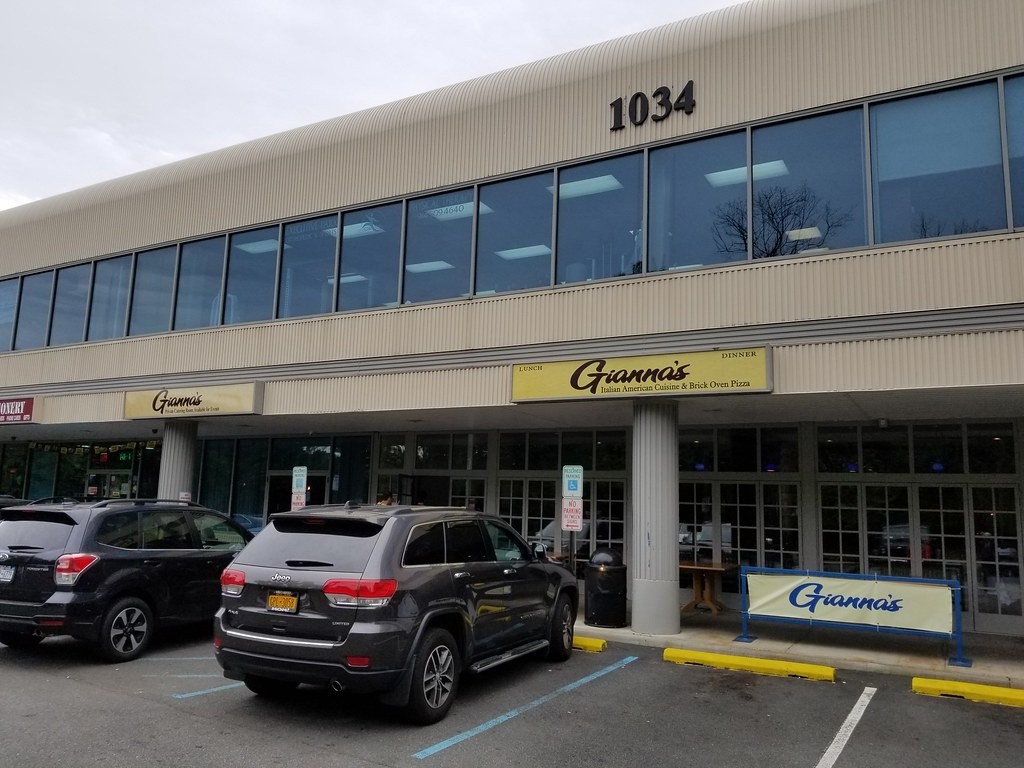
[878,523,933,567]
[530,511,624,576]
[0,496,258,663]
[211,503,580,722]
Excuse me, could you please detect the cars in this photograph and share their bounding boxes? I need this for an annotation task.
[230,513,264,534]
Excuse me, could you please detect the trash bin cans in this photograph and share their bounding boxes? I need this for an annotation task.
[584,547,628,628]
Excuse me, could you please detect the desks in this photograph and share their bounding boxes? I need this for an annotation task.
[678,561,739,617]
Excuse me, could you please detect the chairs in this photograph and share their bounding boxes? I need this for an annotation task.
[664,253,694,268]
[157,521,184,550]
[564,263,587,283]
